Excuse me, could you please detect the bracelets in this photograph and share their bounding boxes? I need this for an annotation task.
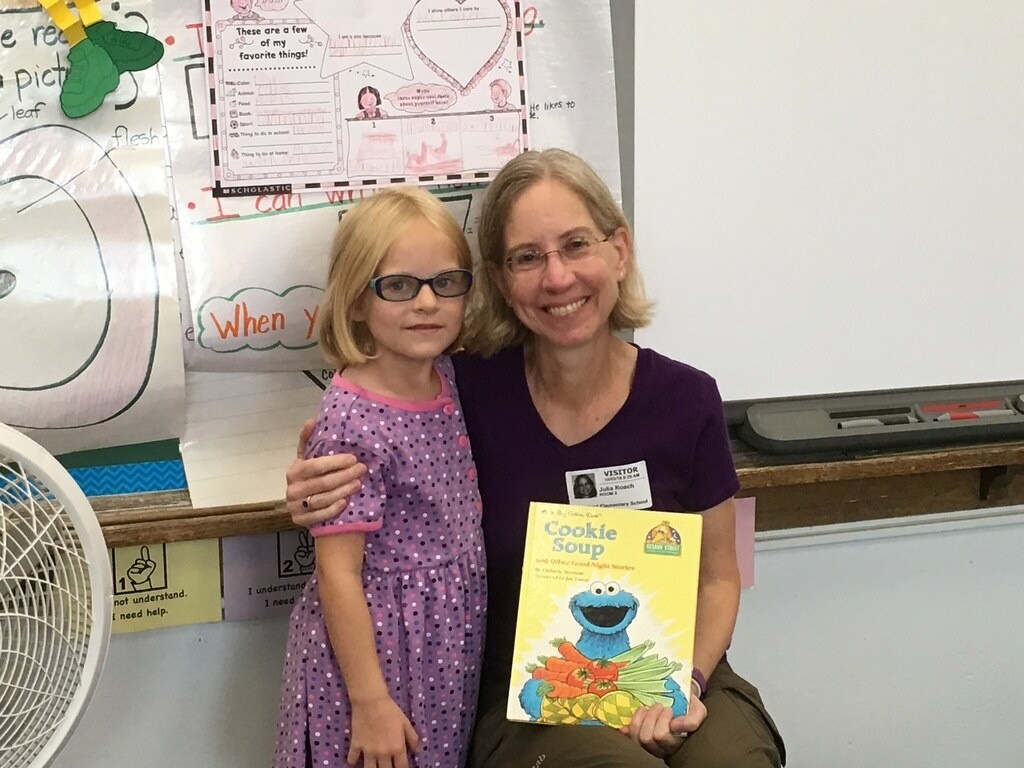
[692,667,706,699]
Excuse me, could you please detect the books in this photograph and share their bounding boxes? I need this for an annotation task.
[507,502,703,737]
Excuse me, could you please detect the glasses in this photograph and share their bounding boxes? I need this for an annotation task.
[503,231,612,274]
[369,269,473,302]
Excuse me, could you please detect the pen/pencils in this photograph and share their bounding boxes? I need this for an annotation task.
[923,401,1005,412]
[837,414,921,429]
[829,406,912,419]
[932,409,1014,422]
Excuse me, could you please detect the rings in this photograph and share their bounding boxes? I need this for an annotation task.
[303,495,314,511]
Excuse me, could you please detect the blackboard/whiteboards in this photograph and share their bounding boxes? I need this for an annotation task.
[609,0,1024,455]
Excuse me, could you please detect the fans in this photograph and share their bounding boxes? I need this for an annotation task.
[0,421,113,768]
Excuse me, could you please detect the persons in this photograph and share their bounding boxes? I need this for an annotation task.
[573,474,597,499]
[273,186,487,768]
[286,149,787,768]
